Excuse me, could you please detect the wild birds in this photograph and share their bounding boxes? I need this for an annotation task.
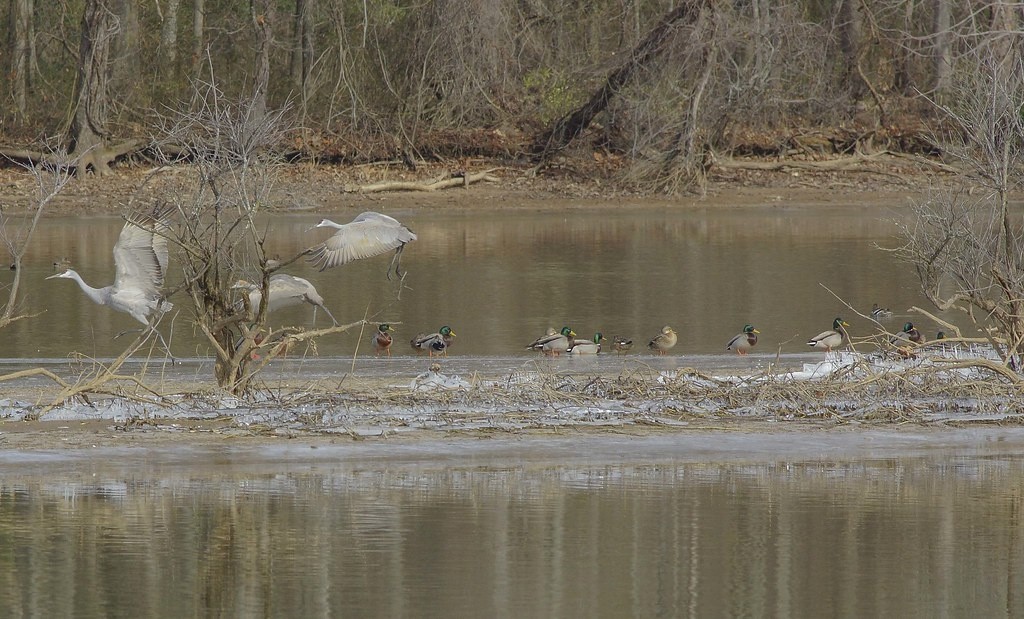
[871,304,894,317]
[890,322,920,347]
[567,332,606,354]
[302,210,418,281]
[726,325,760,355]
[411,333,425,356]
[806,318,850,353]
[930,332,950,348]
[371,324,395,357]
[414,333,447,361]
[647,326,678,356]
[611,335,633,357]
[44,201,181,368]
[231,274,350,335]
[438,326,456,347]
[525,326,577,359]
